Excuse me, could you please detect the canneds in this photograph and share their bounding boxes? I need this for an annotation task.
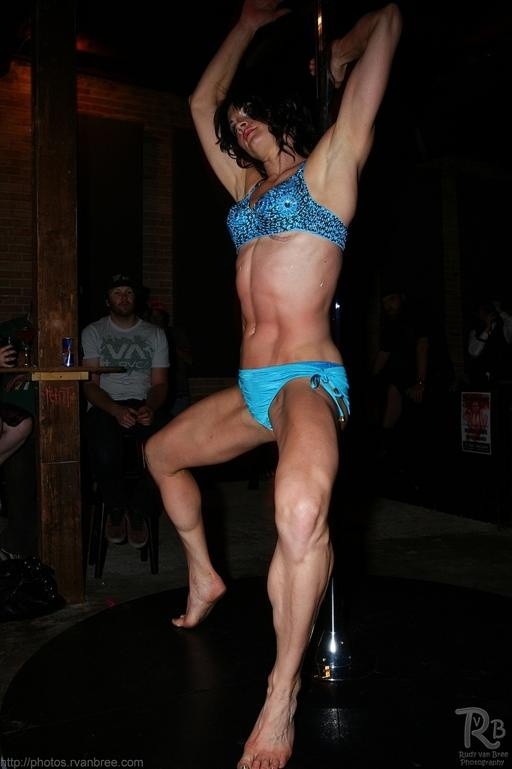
[62,337,73,368]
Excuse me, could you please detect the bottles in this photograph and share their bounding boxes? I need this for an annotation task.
[3,330,18,368]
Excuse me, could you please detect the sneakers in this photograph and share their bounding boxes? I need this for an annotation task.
[125,509,149,548]
[105,512,127,544]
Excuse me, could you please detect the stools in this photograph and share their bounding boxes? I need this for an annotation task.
[84,473,159,584]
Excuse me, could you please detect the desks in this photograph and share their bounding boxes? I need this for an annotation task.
[6,357,136,394]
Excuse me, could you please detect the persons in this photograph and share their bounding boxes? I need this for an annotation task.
[0,342,32,468]
[465,399,488,442]
[79,276,173,551]
[378,279,428,427]
[139,2,404,769]
